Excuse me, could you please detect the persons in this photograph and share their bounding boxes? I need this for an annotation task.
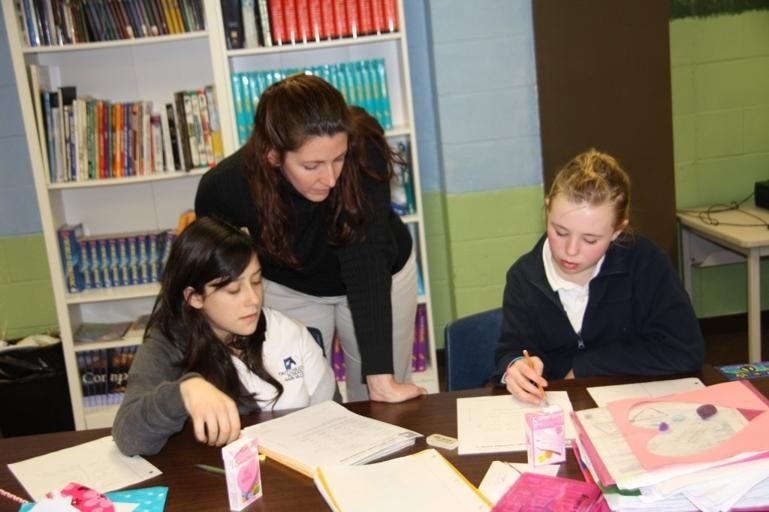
[489,147,703,407]
[110,213,336,458]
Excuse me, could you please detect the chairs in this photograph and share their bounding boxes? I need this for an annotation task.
[443,304,506,391]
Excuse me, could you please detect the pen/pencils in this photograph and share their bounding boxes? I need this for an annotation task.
[260,455,266,461]
[195,464,226,474]
[523,350,550,406]
[0,489,29,504]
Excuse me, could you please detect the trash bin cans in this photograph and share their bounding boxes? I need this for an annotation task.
[0,332,76,438]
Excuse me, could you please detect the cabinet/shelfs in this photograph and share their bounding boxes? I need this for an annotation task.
[1,0,232,432]
[215,0,441,405]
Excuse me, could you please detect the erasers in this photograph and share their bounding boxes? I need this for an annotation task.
[426,433,459,450]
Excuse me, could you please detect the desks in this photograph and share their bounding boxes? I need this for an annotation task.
[0,378,769,512]
[677,203,768,366]
